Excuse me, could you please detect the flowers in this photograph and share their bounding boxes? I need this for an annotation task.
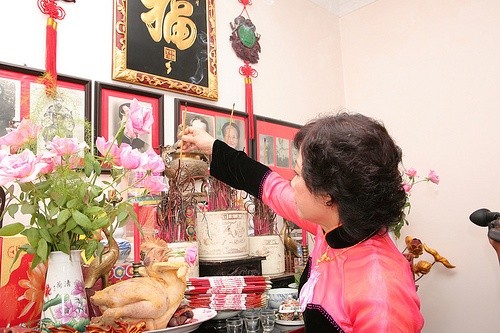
[0,97,170,272]
[390,167,440,242]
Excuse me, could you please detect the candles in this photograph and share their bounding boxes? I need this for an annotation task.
[133,190,140,264]
[302,228,306,247]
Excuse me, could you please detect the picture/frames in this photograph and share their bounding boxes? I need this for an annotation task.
[254,115,310,274]
[0,61,93,173]
[174,98,254,159]
[95,81,164,174]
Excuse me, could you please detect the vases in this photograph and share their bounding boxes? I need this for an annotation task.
[40,250,90,333]
[167,239,199,282]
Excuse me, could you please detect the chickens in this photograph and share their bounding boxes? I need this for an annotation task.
[89,235,187,330]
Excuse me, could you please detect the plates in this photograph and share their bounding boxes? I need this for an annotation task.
[213,310,242,319]
[142,308,217,333]
[288,283,297,289]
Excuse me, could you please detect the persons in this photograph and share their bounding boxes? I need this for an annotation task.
[189,117,208,133]
[119,103,145,149]
[222,122,240,148]
[179,112,424,333]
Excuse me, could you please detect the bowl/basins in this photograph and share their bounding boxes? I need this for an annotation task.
[267,288,298,308]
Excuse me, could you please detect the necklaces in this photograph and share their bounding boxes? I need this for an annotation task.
[317,229,376,263]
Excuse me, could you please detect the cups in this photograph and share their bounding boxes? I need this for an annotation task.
[260,309,276,333]
[244,316,260,333]
[279,309,304,322]
[226,317,243,333]
